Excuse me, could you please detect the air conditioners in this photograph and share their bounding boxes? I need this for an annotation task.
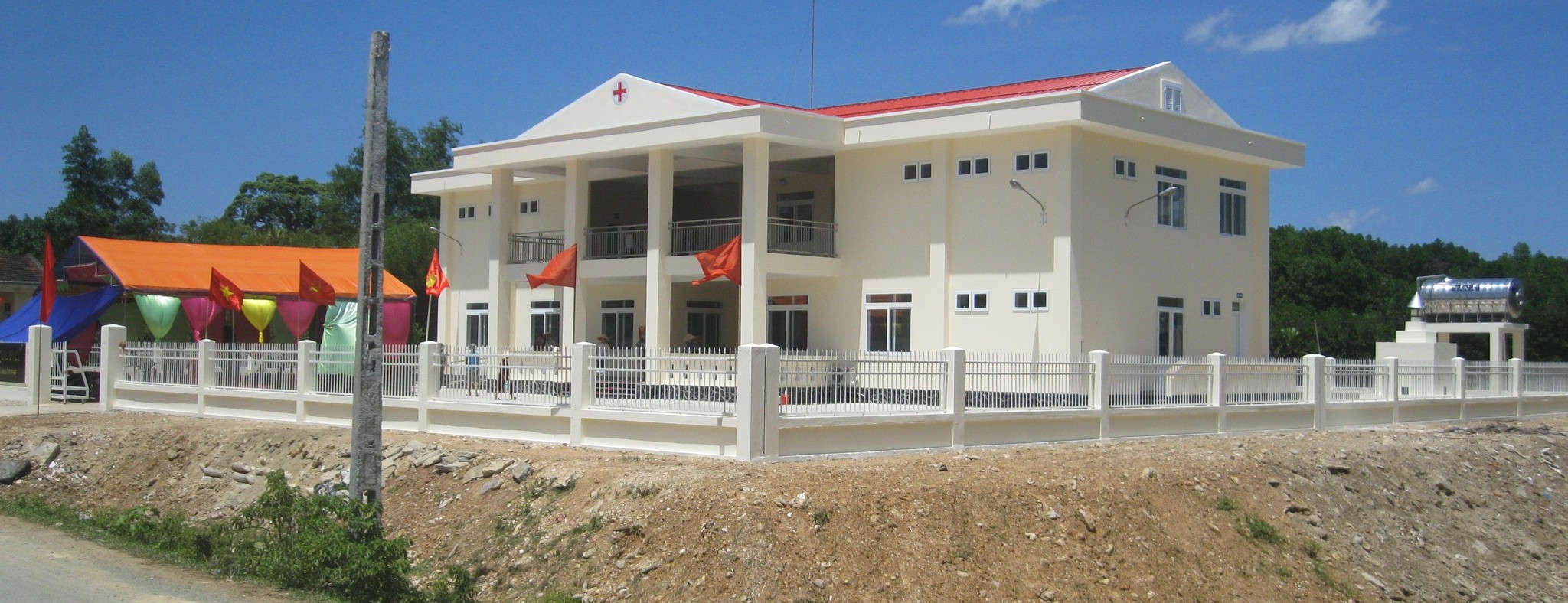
[829,366,855,388]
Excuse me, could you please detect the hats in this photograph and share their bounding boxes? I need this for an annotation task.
[469,343,476,350]
[684,333,695,341]
[502,351,512,359]
[597,334,609,342]
[436,343,445,351]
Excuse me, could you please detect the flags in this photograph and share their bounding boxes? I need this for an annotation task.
[691,235,741,286]
[40,234,56,323]
[208,267,244,312]
[425,249,450,298]
[526,243,577,289]
[299,262,336,306]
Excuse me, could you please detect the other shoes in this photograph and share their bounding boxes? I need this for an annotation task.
[494,397,501,400]
[466,394,471,396]
[511,397,517,400]
[476,394,478,396]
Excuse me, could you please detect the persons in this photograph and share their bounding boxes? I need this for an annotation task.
[636,333,645,381]
[534,331,557,351]
[596,334,610,379]
[494,352,518,400]
[438,343,446,388]
[683,333,699,353]
[466,343,479,397]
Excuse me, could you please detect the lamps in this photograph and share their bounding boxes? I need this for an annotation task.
[1124,187,1178,227]
[428,226,463,255]
[1009,179,1046,226]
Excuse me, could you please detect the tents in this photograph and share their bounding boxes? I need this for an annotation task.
[37,237,416,371]
